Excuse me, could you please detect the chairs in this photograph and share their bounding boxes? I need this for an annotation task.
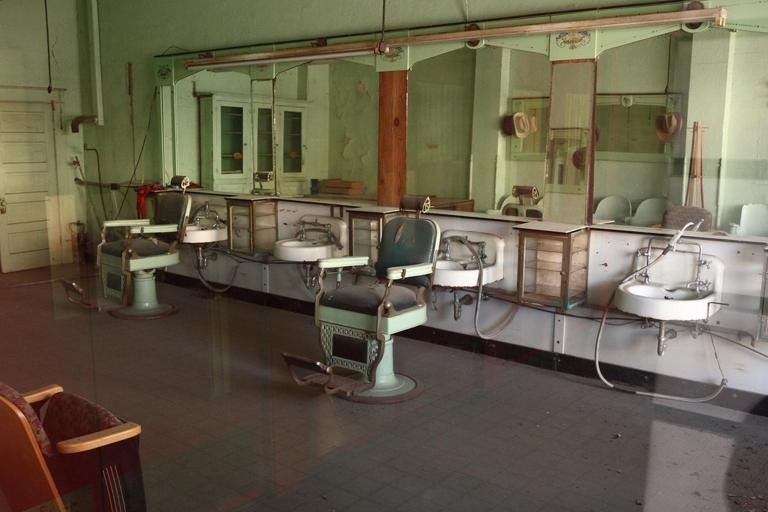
[0,381,142,510]
[281,217,442,404]
[58,194,189,319]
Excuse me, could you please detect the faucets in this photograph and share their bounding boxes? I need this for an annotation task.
[472,255,483,261]
[205,202,210,216]
[686,280,699,289]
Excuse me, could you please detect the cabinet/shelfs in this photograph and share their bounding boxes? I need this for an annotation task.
[513,221,590,310]
[346,207,401,268]
[224,196,278,256]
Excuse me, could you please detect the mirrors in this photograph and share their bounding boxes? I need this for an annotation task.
[405,10,767,243]
[156,51,379,205]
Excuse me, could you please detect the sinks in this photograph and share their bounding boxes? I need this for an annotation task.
[616,280,715,321]
[182,225,227,244]
[274,238,335,262]
[434,257,496,288]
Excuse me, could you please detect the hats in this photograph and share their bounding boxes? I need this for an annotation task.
[512,111,537,138]
[655,112,683,144]
